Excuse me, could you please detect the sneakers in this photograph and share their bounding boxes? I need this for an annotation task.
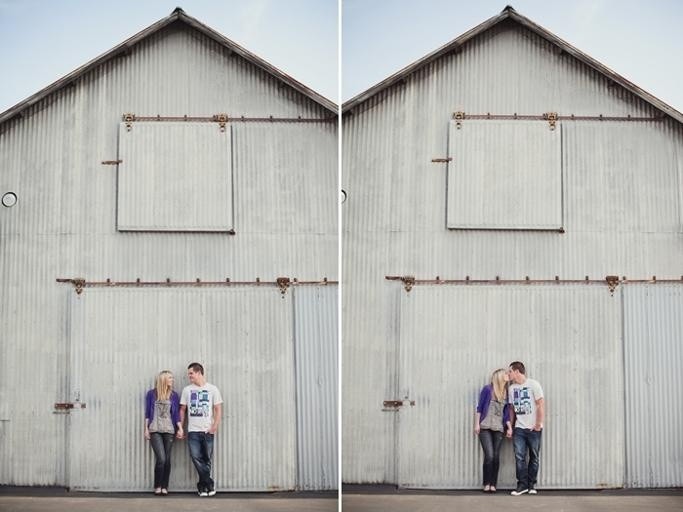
[197,489,217,496]
[154,485,168,494]
[528,482,537,494]
[484,482,496,491]
[511,485,529,496]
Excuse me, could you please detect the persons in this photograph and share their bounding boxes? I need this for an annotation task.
[144,370,184,494]
[473,369,513,494]
[506,361,544,496]
[177,362,222,498]
